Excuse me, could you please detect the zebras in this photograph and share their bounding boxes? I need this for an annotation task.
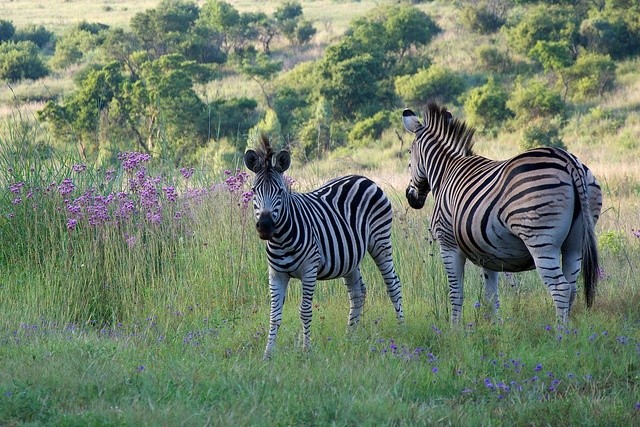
[243,131,406,362]
[402,96,603,329]
